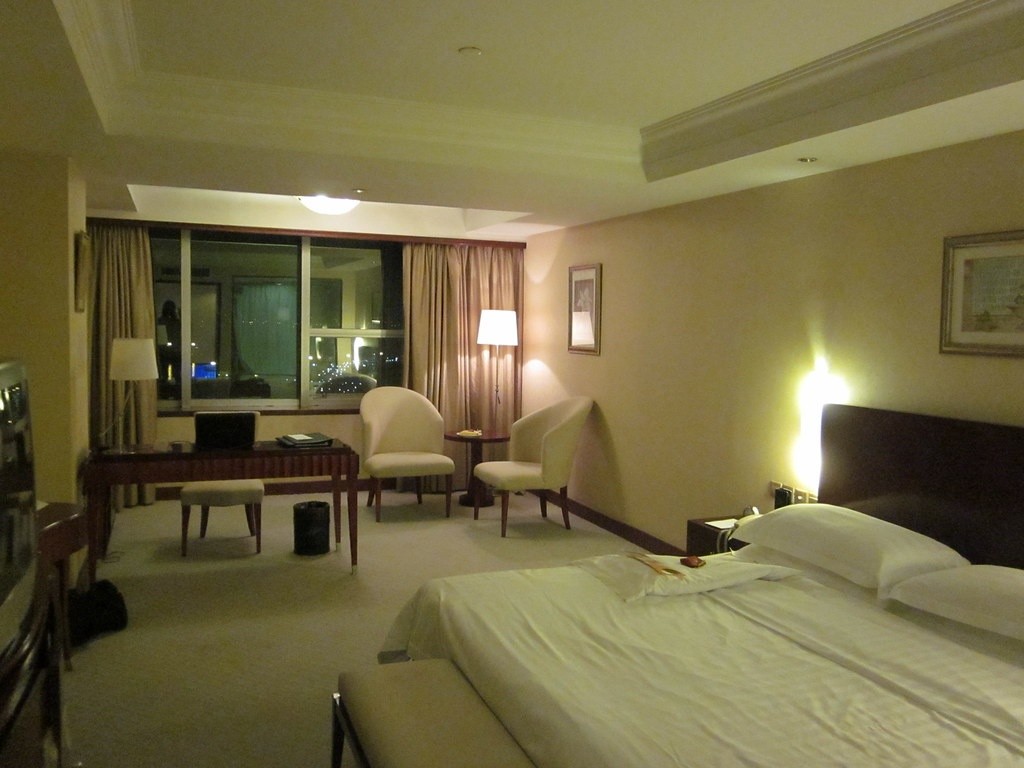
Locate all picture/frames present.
[939,230,1023,358]
[566,265,602,355]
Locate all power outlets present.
[770,481,793,501]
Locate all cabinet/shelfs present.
[0,502,85,768]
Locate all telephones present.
[733,513,764,527]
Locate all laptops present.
[194,413,255,449]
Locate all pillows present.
[885,557,1023,642]
[728,508,972,602]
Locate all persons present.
[158,300,181,384]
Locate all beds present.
[373,402,1024,768]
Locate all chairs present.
[360,386,455,523]
[471,399,596,539]
[177,410,263,557]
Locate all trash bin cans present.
[292,500,330,556]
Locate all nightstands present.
[686,515,748,561]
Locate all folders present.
[283,432,334,447]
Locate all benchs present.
[331,657,531,768]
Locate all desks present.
[444,429,511,507]
[77,438,357,586]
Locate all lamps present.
[88,338,158,454]
[477,309,518,495]
